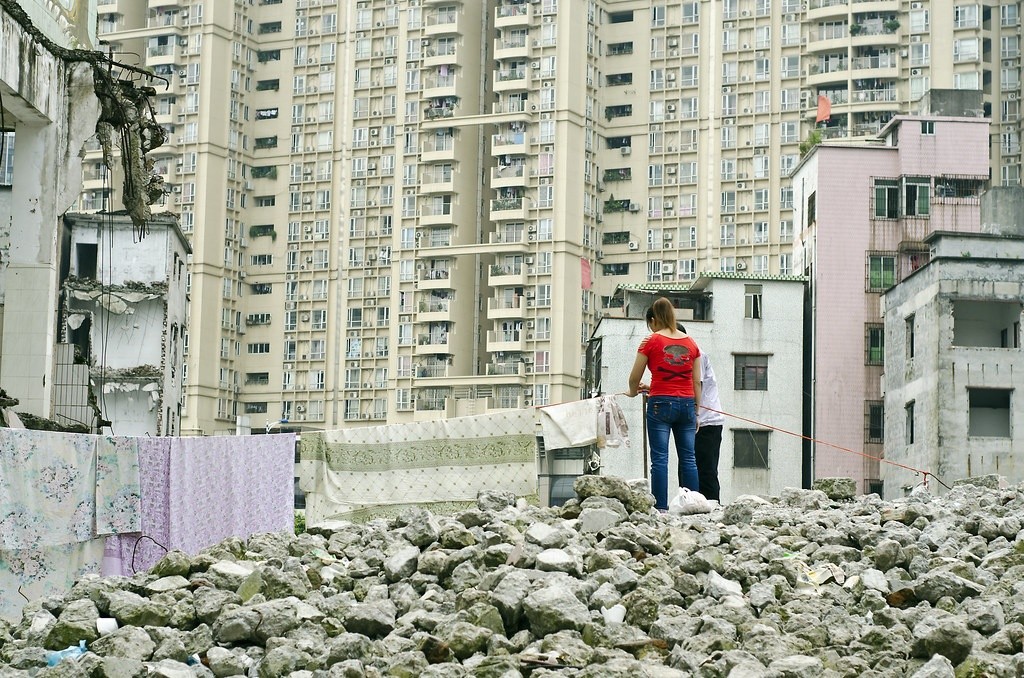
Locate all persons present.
[621,297,702,514]
[637,322,725,505]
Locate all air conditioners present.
[128,9,1023,422]
